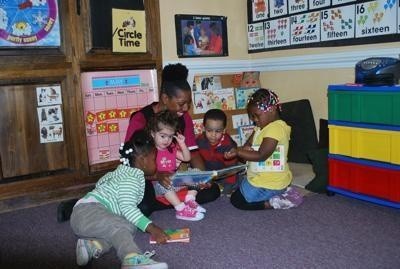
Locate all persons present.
[55,62,220,224]
[145,110,207,221]
[224,88,302,210]
[194,109,240,194]
[70,128,172,269]
[182,20,223,56]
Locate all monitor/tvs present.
[175,13,229,58]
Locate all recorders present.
[354,57,400,86]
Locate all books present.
[188,71,260,147]
[149,228,190,244]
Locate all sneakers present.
[122,255,168,268]
[77,239,98,265]
[184,199,206,213]
[176,207,204,221]
[57,198,77,222]
[269,187,302,209]
